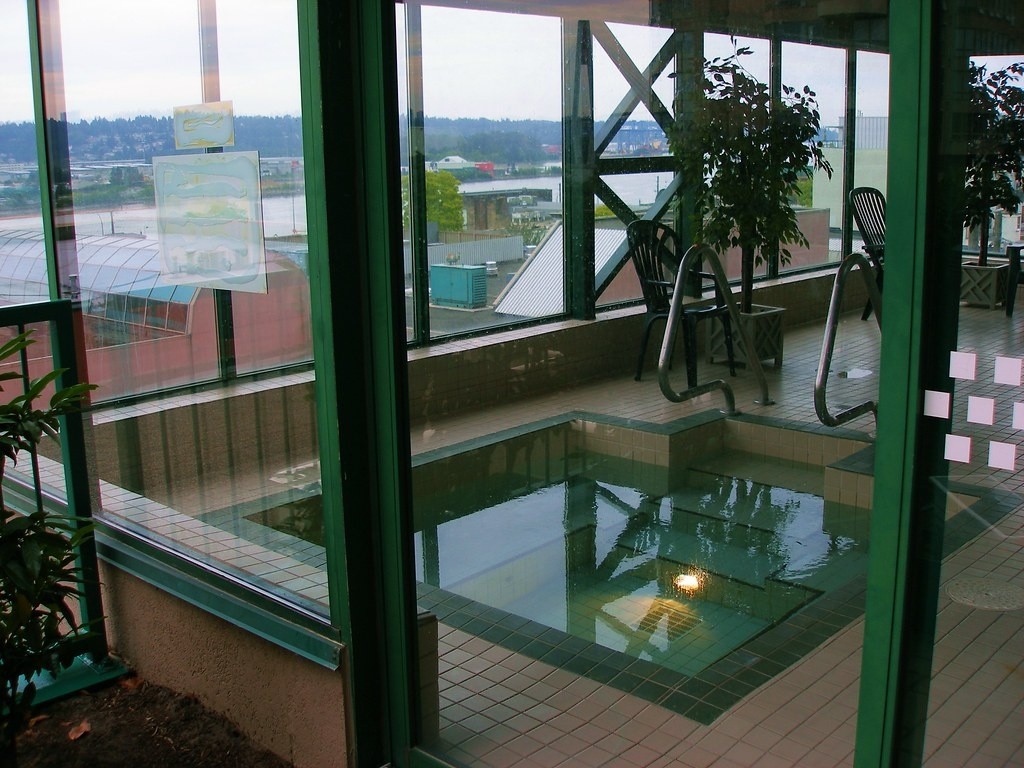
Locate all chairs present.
[1005,245,1024,318]
[848,186,886,320]
[626,219,738,390]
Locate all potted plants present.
[959,61,1024,311]
[666,29,835,371]
